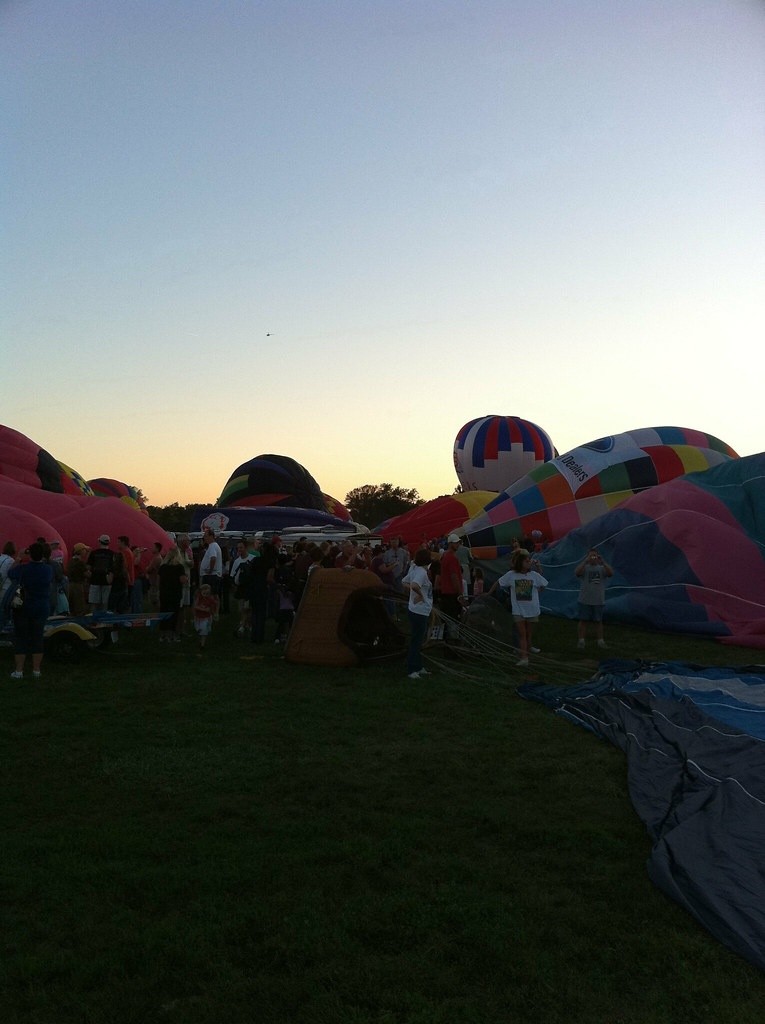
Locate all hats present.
[447,534,462,543]
[272,537,282,542]
[74,543,90,554]
[97,535,111,544]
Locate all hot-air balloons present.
[214,415,765,649]
[0,425,177,582]
[285,566,765,968]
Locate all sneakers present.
[417,667,433,675]
[408,672,421,679]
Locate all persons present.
[575,549,614,647]
[1,528,549,678]
[440,533,469,651]
[7,543,54,678]
[477,549,548,666]
[400,549,434,679]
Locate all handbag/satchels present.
[10,584,24,609]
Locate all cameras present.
[143,548,147,551]
[591,556,598,560]
[25,548,30,554]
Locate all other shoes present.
[249,627,252,631]
[10,670,24,678]
[596,639,607,649]
[577,638,585,648]
[528,647,541,654]
[274,639,279,646]
[515,657,529,666]
[237,626,245,633]
[32,670,42,676]
